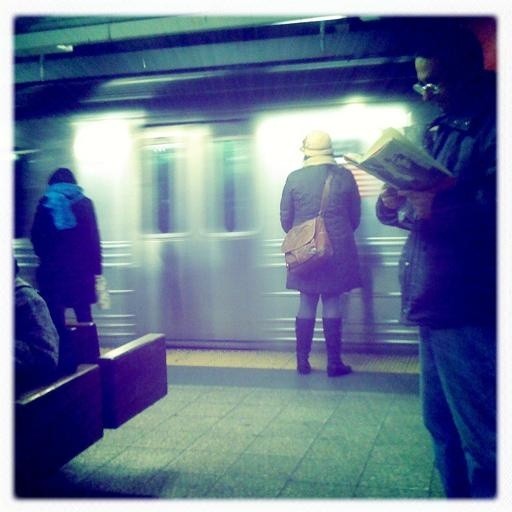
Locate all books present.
[343,126,457,195]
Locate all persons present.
[14,258,61,394]
[27,166,102,340]
[373,45,494,500]
[278,129,363,377]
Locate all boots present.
[323,317,351,377]
[295,318,315,374]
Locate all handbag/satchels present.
[281,217,333,275]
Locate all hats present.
[300,133,332,155]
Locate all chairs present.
[13,319,168,476]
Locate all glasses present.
[413,83,441,95]
